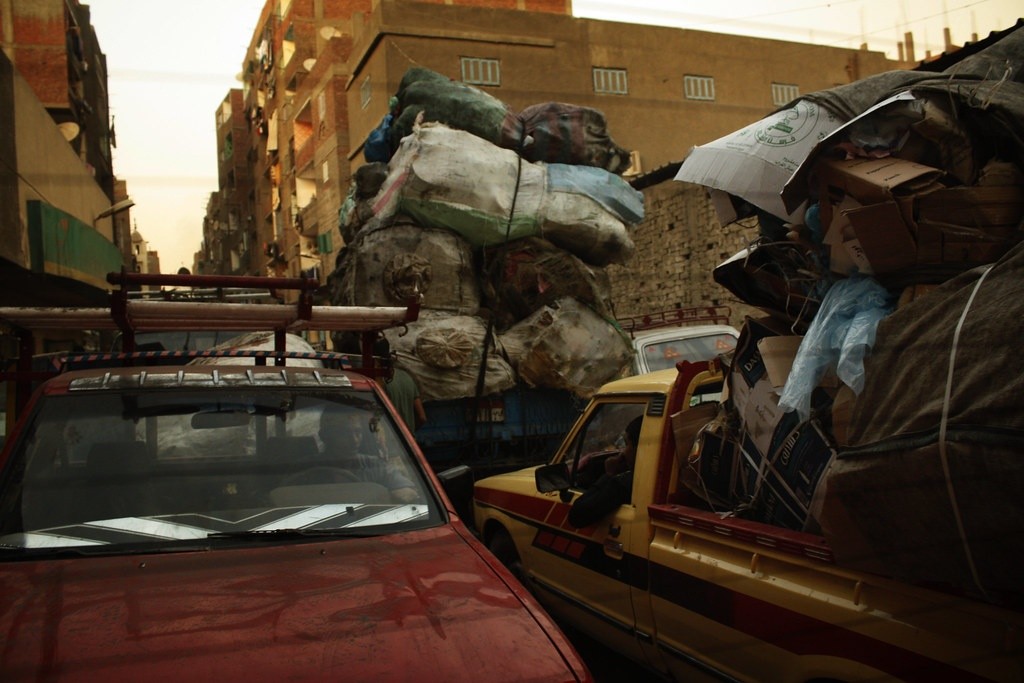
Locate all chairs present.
[241,435,319,510]
[69,440,172,524]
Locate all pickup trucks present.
[0,266,597,683]
[464,360,1023,681]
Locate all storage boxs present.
[666,70,1024,541]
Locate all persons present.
[290,402,418,505]
[568,416,644,529]
